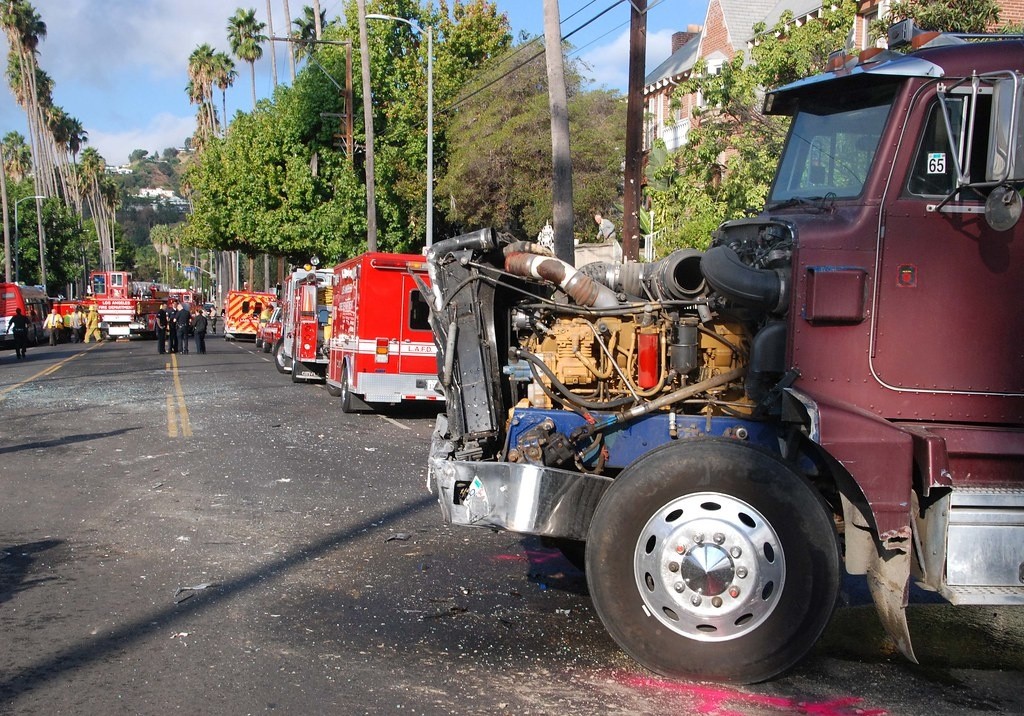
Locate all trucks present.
[426,20,1024,685]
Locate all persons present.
[6,308,32,359]
[155,299,217,355]
[43,304,102,347]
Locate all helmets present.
[89,305,95,310]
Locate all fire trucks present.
[274,252,447,413]
[220,291,278,342]
[0,271,199,346]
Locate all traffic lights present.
[177,262,182,272]
[157,272,164,284]
[214,279,216,287]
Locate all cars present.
[254,307,282,356]
[200,302,215,317]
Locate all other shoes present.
[22,351,25,357]
[160,350,206,354]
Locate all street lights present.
[365,14,433,248]
[15,196,46,281]
